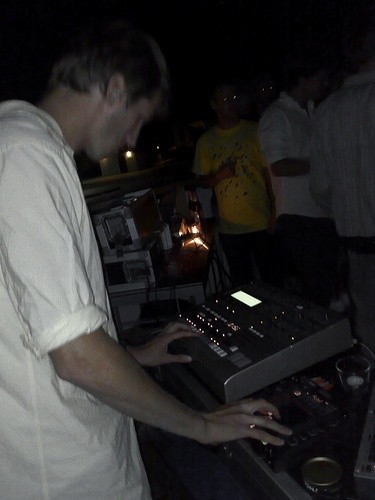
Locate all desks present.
[106,217,233,334]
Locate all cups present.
[334,356,371,400]
[301,456,343,498]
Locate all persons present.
[166,18,374,412]
[2,0,293,500]
